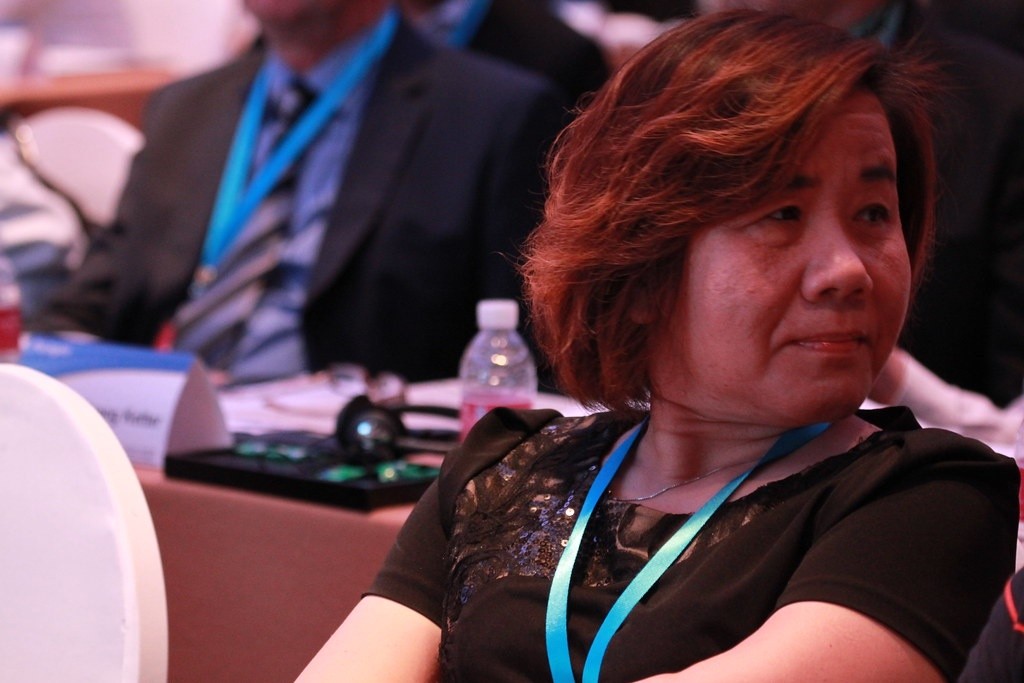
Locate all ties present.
[173,74,322,371]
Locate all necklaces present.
[612,456,760,502]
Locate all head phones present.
[335,395,463,466]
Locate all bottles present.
[458,298,537,444]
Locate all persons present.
[699,0,1024,451]
[47,1,700,385]
[291,9,1021,683]
